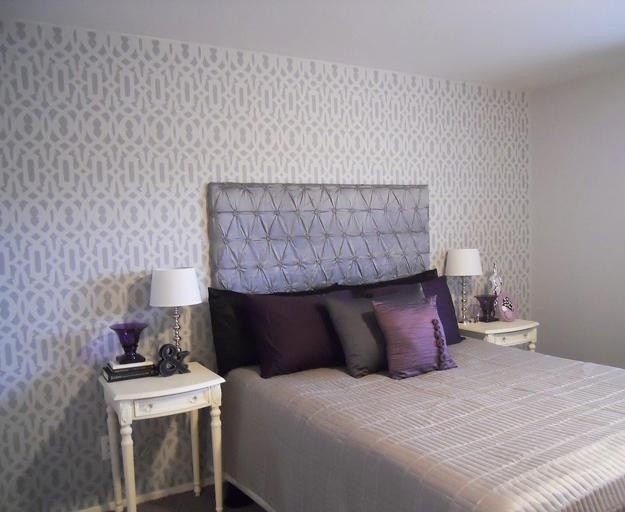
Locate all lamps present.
[148,266,210,371]
[443,247,486,326]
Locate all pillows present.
[207,267,468,382]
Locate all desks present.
[95,359,228,471]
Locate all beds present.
[204,181,625,471]
